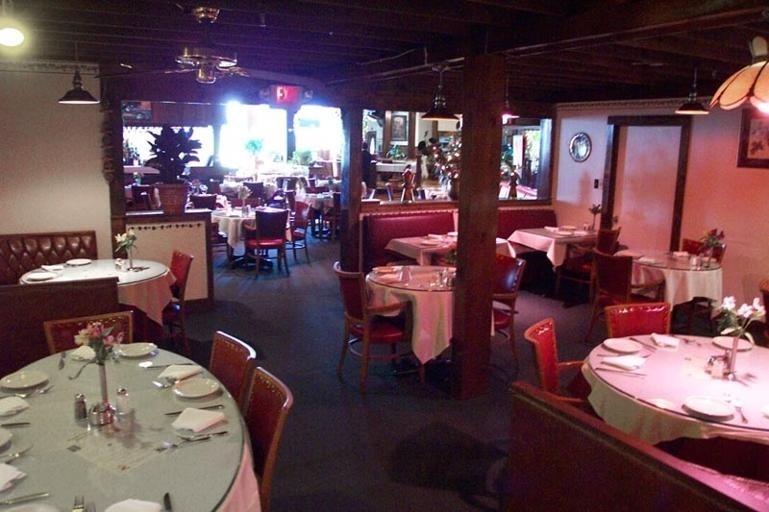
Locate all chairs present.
[126,157,519,281]
[1,226,195,358]
[209,328,257,417]
[524,317,588,408]
[329,200,725,386]
[241,365,294,512]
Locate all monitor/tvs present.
[270,83,304,109]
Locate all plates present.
[28,273,55,280]
[119,341,158,358]
[173,378,220,398]
[67,259,92,266]
[603,336,643,353]
[713,335,752,351]
[372,266,400,274]
[102,499,158,512]
[0,370,58,511]
[682,395,736,419]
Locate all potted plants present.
[144,121,203,215]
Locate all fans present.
[95,5,327,90]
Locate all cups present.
[428,269,454,290]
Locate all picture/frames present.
[389,115,407,141]
[737,107,769,168]
[567,130,593,162]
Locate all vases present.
[727,336,741,381]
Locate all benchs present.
[507,379,768,510]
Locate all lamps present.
[707,34,769,116]
[501,74,521,127]
[58,35,101,106]
[676,68,709,116]
[420,62,459,124]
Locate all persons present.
[361,142,371,187]
[417,137,444,162]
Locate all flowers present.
[713,292,767,341]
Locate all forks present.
[171,429,227,441]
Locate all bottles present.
[399,266,411,284]
[73,393,87,421]
[114,387,132,416]
[115,257,122,271]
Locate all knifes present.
[142,363,193,369]
[57,352,67,369]
[163,492,172,511]
[595,368,647,377]
[164,404,224,416]
